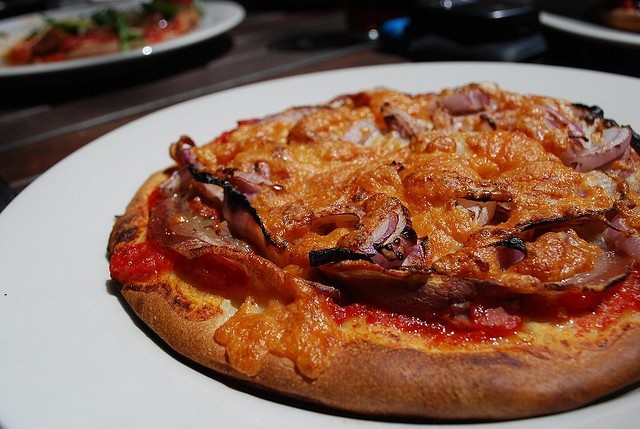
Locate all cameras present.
[380,0,549,65]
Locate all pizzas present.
[107,81,637,420]
[0,0,204,64]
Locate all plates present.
[0,0,247,75]
[0,60,640,427]
